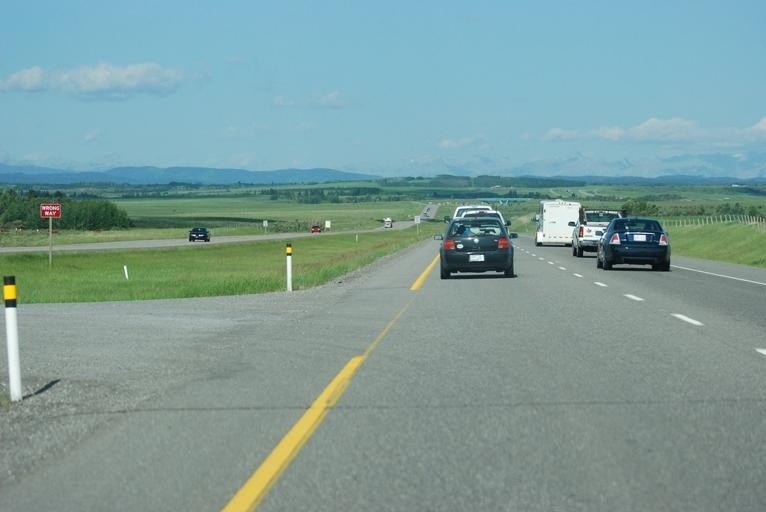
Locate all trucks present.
[385,218,392,228]
[534,200,581,246]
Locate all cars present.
[311,226,321,233]
[597,217,670,269]
[434,205,518,278]
[189,228,210,242]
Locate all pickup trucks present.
[572,210,622,256]
